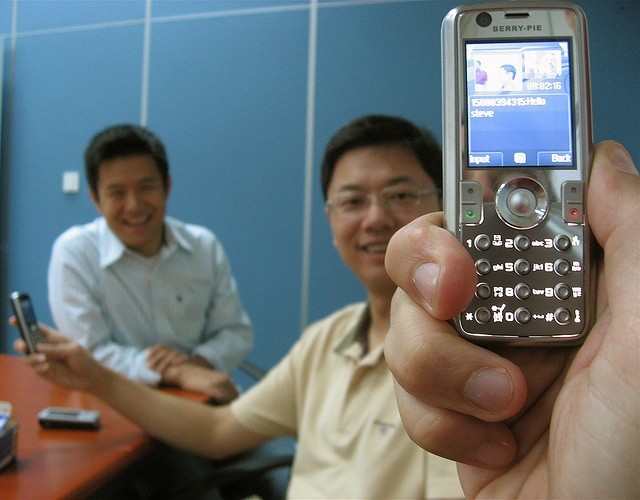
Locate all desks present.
[0,351,213,500]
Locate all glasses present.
[326,187,438,217]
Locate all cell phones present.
[440,0,598,348]
[10,290,46,354]
[37,407,102,430]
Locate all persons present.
[499,64,518,91]
[9,114,465,500]
[383,139,640,499]
[46,122,298,500]
[475,60,487,85]
